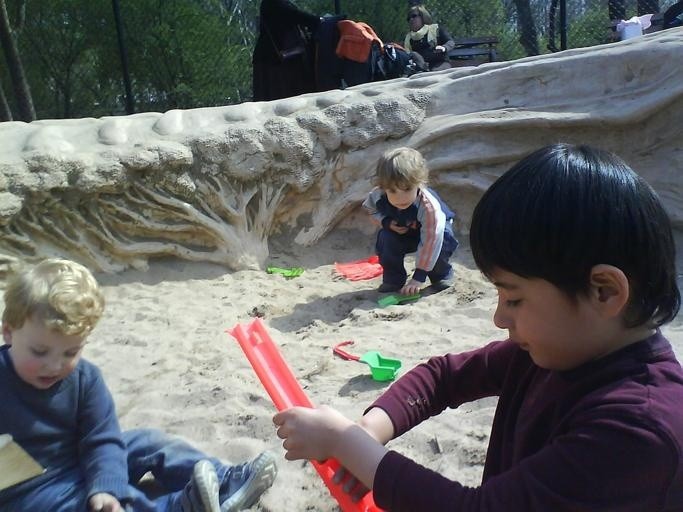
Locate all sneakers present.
[377,274,407,292]
[434,271,455,288]
[181,459,220,511]
[220,451,277,511]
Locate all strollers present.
[320,12,428,85]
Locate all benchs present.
[605,13,665,42]
[445,36,500,68]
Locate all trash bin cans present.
[620,19,643,40]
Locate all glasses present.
[407,13,421,21]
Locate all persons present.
[403,3,455,74]
[359,143,459,293]
[270,141,680,511]
[1,258,279,512]
